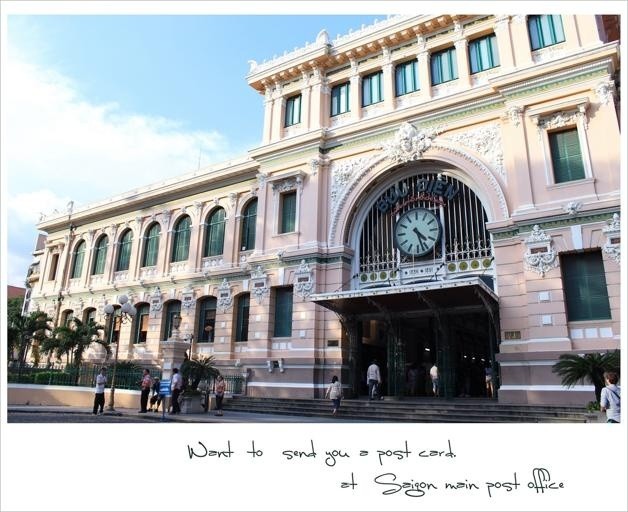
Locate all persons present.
[366,359,382,401]
[215,375,225,416]
[382,361,495,398]
[91,367,107,416]
[147,376,165,412]
[137,369,151,413]
[324,375,343,417]
[599,371,619,423]
[170,368,182,414]
[197,377,209,412]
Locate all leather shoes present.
[138,409,147,413]
[169,410,181,415]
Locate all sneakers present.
[214,413,224,416]
[92,413,104,416]
[147,409,159,412]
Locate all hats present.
[154,376,160,381]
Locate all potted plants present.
[550,349,620,423]
[178,352,220,413]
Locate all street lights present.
[104,295,137,413]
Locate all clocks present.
[394,208,441,258]
